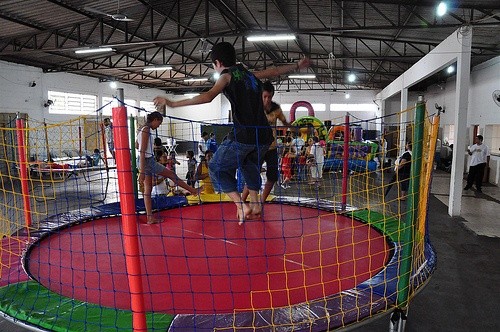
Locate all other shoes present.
[286,186,291,188]
[477,188,482,192]
[281,185,286,189]
[464,185,471,190]
[400,197,406,200]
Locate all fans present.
[492,90,500,107]
[83,0,135,21]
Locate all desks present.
[163,144,179,156]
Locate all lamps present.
[73,48,117,54]
[142,66,175,71]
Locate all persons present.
[394,142,412,201]
[464,135,491,193]
[237,80,300,217]
[275,131,324,190]
[137,110,218,224]
[83,149,105,167]
[100,118,115,159]
[152,39,314,225]
